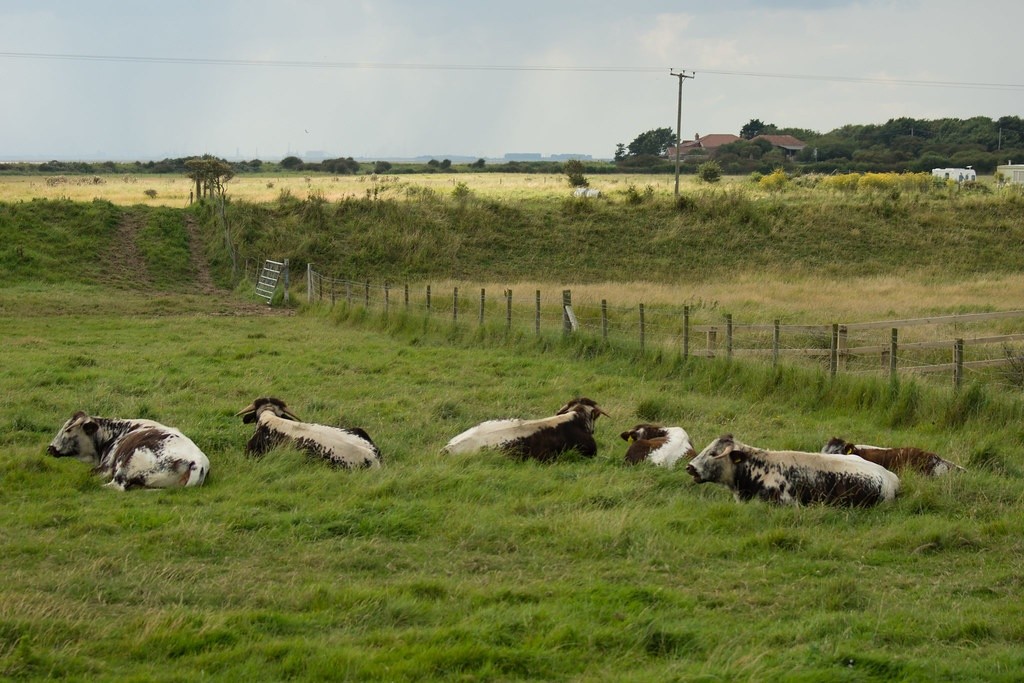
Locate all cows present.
[47,411,210,491]
[685,433,902,511]
[819,436,967,477]
[440,396,610,466]
[236,396,384,474]
[619,422,696,470]
